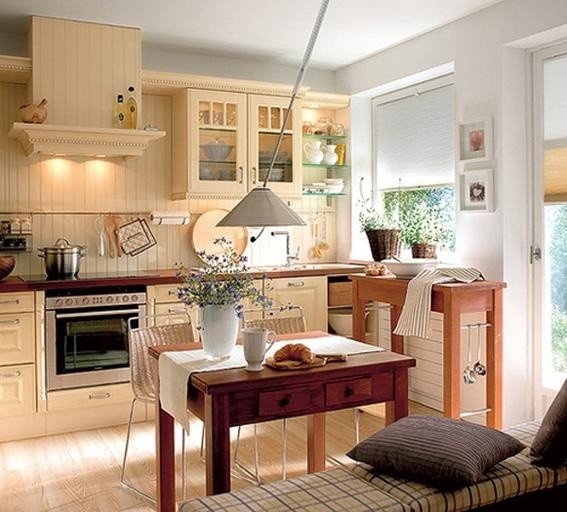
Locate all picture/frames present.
[455,117,493,164]
[456,169,494,213]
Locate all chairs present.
[120,311,261,507]
[235,305,359,482]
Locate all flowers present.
[167,237,296,319]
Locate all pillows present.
[344,413,529,488]
[529,378,567,468]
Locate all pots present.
[37,238,88,281]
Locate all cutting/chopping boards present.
[265,353,347,372]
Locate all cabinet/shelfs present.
[264,276,328,331]
[171,88,303,201]
[0,292,37,417]
[147,280,264,339]
[47,383,146,436]
[303,135,349,196]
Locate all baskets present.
[411,243,436,259]
[366,228,401,262]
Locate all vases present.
[198,306,238,361]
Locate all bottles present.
[10,218,21,236]
[337,143,346,166]
[21,217,32,235]
[0,220,10,236]
[124,85,139,131]
[113,94,127,129]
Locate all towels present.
[393,265,487,341]
[265,344,347,372]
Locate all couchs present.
[178,419,567,511]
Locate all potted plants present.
[356,176,404,260]
[401,194,455,258]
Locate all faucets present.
[272,229,300,264]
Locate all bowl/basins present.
[200,143,236,160]
[259,168,284,181]
[328,308,370,337]
[324,178,345,195]
[380,258,441,279]
[1,255,17,282]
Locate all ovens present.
[44,285,147,392]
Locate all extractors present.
[8,14,167,161]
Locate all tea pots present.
[321,144,339,165]
[303,141,323,165]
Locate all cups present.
[199,166,216,180]
[203,110,220,124]
[218,168,236,180]
[214,111,229,125]
[242,328,276,372]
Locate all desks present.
[349,274,507,431]
[148,330,416,512]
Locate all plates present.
[258,151,289,161]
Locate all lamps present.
[213,1,330,227]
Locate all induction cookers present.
[18,268,161,283]
